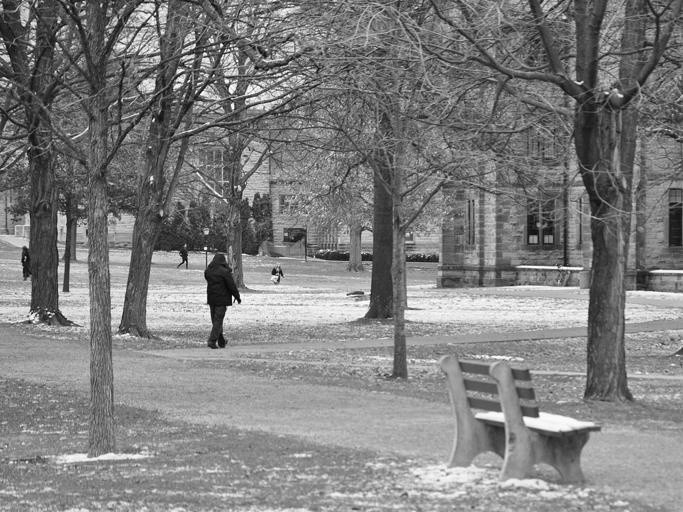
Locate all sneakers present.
[208,342,218,349]
[219,337,229,348]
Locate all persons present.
[203,252,240,349]
[178,245,188,269]
[271,264,285,283]
[21,246,31,280]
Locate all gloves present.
[232,295,242,305]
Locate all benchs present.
[436,353,601,484]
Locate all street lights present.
[202,226,210,267]
[303,222,308,261]
